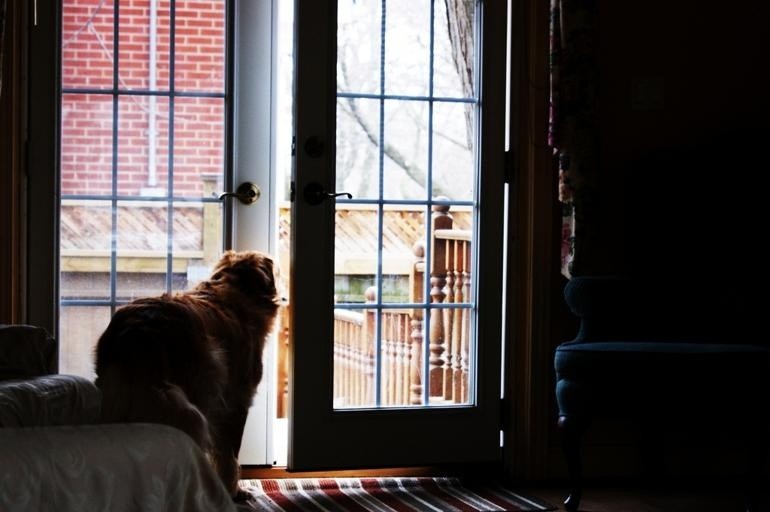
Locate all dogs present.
[90,249,280,504]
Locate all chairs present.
[550,276,767,511]
